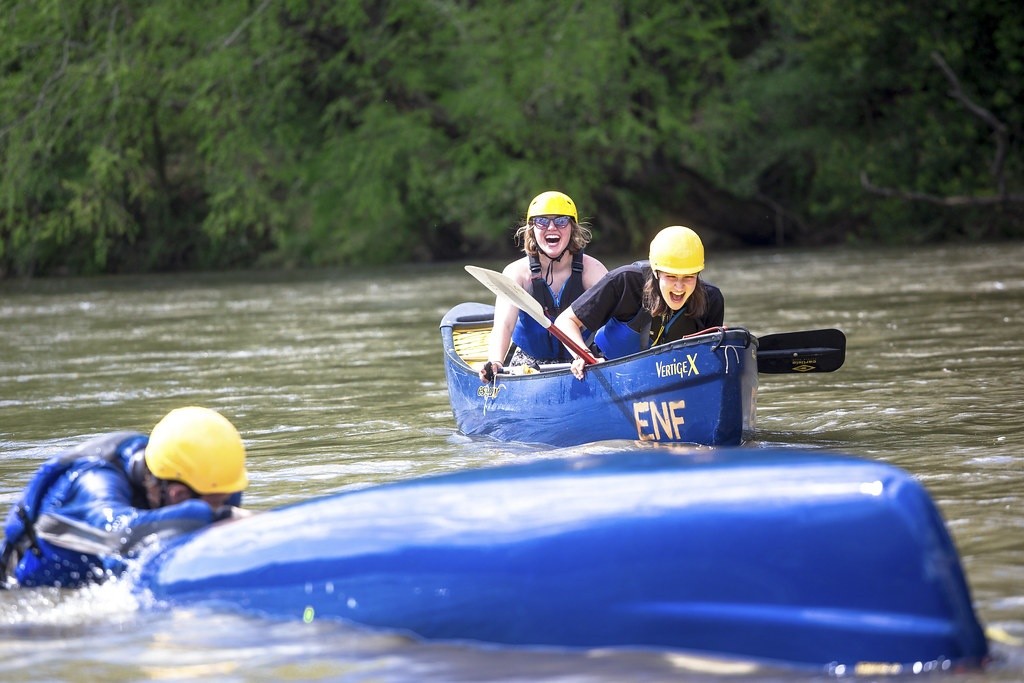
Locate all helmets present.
[144,406,249,496]
[649,226,705,279]
[527,191,578,226]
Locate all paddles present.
[463,262,598,366]
[497,327,848,376]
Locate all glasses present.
[532,216,571,231]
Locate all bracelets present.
[576,349,591,360]
[493,361,503,367]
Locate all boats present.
[439,301,760,449]
[118,444,992,669]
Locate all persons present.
[554,225,725,379]
[6,406,252,588]
[479,191,609,382]
